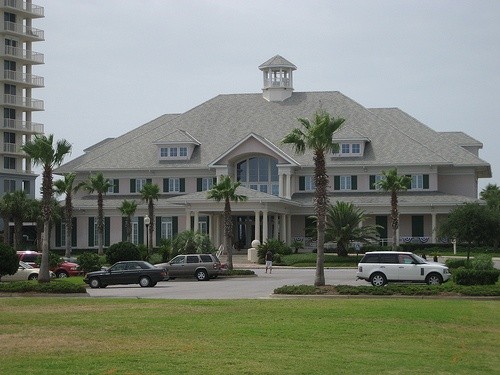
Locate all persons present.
[265,249,273,274]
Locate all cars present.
[1,261,56,281]
[83,261,168,288]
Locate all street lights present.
[144,215,150,249]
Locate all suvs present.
[153,254,221,281]
[356,252,452,287]
[16,251,81,278]
